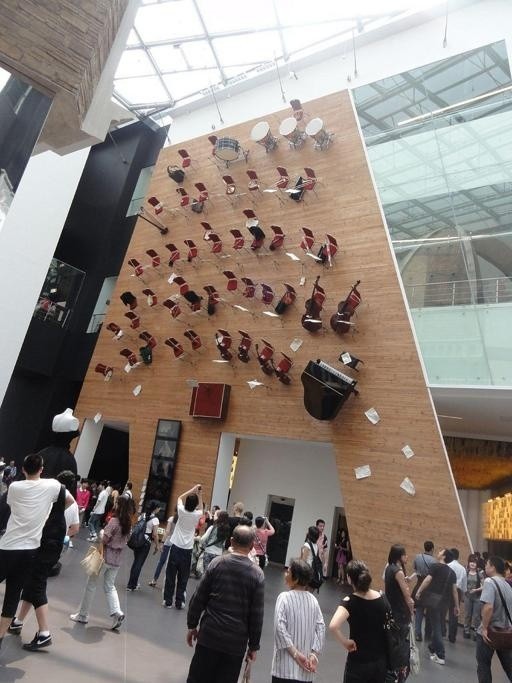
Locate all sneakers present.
[429,654,445,667]
[22,631,51,650]
[161,598,173,609]
[126,583,140,592]
[175,600,185,610]
[6,617,22,635]
[70,612,89,624]
[85,521,97,542]
[111,611,125,629]
[416,632,478,641]
[68,541,74,548]
[149,579,157,587]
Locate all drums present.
[279,117,303,149]
[215,139,239,160]
[252,122,277,151]
[306,118,328,146]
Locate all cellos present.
[301,276,322,331]
[271,358,292,385]
[237,340,249,363]
[330,280,360,333]
[215,334,232,361]
[255,344,273,375]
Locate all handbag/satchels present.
[264,552,271,567]
[409,646,419,677]
[80,544,105,581]
[196,551,203,574]
[486,626,512,651]
[419,591,445,609]
[38,502,66,567]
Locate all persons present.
[301,519,349,584]
[272,557,326,682]
[186,523,266,682]
[1,455,134,649]
[328,540,512,682]
[126,484,275,608]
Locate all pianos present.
[301,359,359,421]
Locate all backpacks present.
[127,513,158,552]
[307,542,323,588]
[384,618,413,670]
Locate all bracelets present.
[293,649,297,658]
[308,652,319,662]
